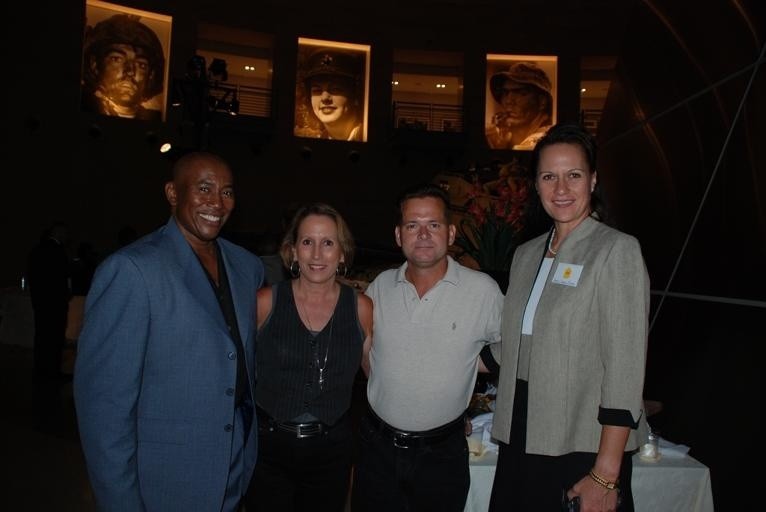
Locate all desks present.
[464,436,715,512]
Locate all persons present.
[255,199,306,287]
[479,123,653,512]
[26,218,164,434]
[350,183,507,512]
[252,200,375,511]
[297,45,369,141]
[485,61,556,154]
[85,9,166,124]
[72,149,364,509]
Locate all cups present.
[640,435,659,457]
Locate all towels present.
[658,437,690,459]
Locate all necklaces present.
[296,273,337,392]
[548,226,560,256]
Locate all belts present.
[261,410,330,440]
[366,402,463,454]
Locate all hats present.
[298,50,368,91]
[489,64,554,116]
[59,17,164,82]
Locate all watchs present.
[587,467,621,492]
